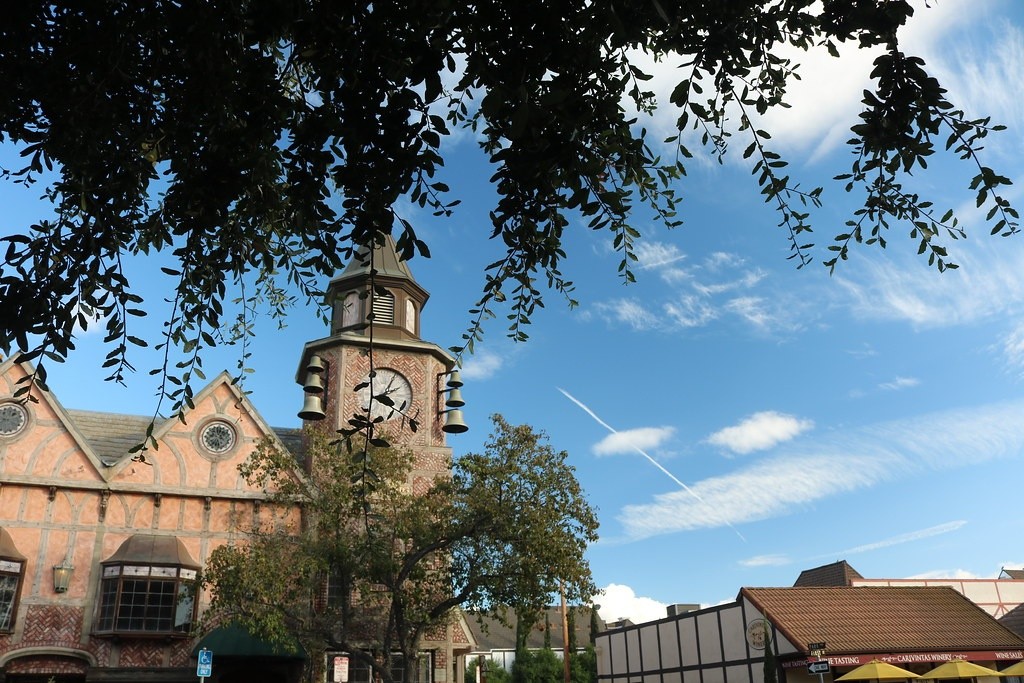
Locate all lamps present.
[53,553,76,592]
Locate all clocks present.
[359,364,416,422]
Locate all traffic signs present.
[806,660,831,676]
